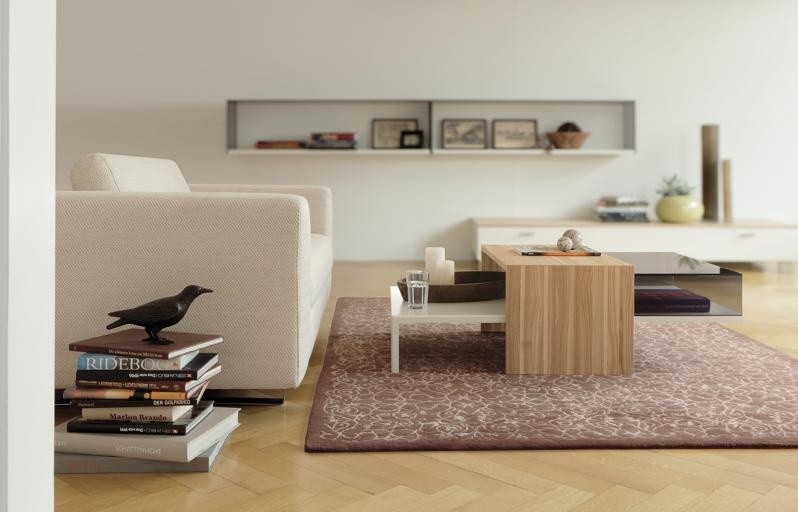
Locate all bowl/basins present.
[544,132,591,149]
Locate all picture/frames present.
[370,119,537,149]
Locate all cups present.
[405,270,429,313]
[425,246,455,286]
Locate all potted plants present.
[654,172,705,222]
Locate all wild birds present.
[106,285,213,344]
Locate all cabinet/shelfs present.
[472,220,798,275]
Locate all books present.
[54,329,242,473]
[515,246,601,256]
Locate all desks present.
[390,243,742,378]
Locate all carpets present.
[306,295,798,449]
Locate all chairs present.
[55,153,335,407]
